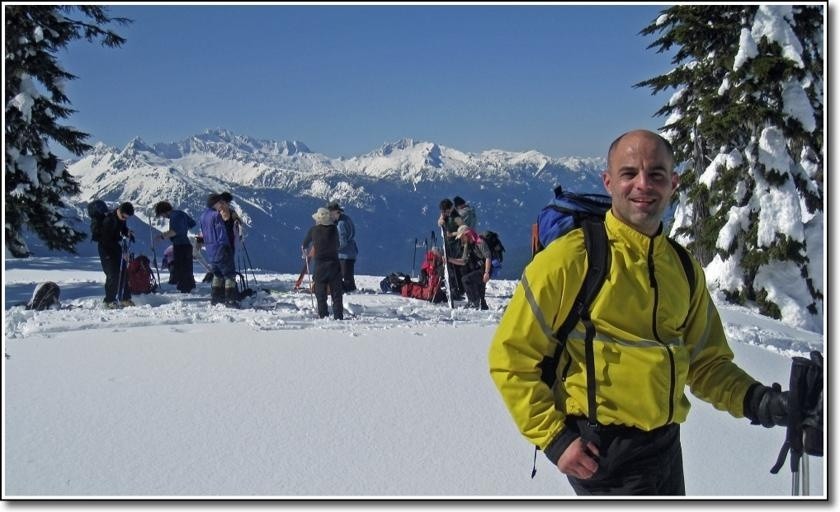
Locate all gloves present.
[743,380,793,426]
[800,348,824,458]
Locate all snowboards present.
[440,223,453,309]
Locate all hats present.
[312,207,332,226]
[207,194,224,208]
[454,196,465,207]
[327,203,344,212]
[455,225,471,240]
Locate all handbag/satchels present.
[379,249,447,303]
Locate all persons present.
[301,207,345,320]
[436,198,463,301]
[89,188,241,309]
[486,129,793,494]
[441,224,490,310]
[453,197,476,226]
[326,202,357,290]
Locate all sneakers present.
[108,300,136,309]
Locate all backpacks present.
[25,280,61,311]
[477,231,506,262]
[126,252,158,294]
[530,184,612,266]
[87,200,111,242]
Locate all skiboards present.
[115,231,136,308]
[294,256,311,294]
[429,231,438,301]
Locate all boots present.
[211,286,240,309]
